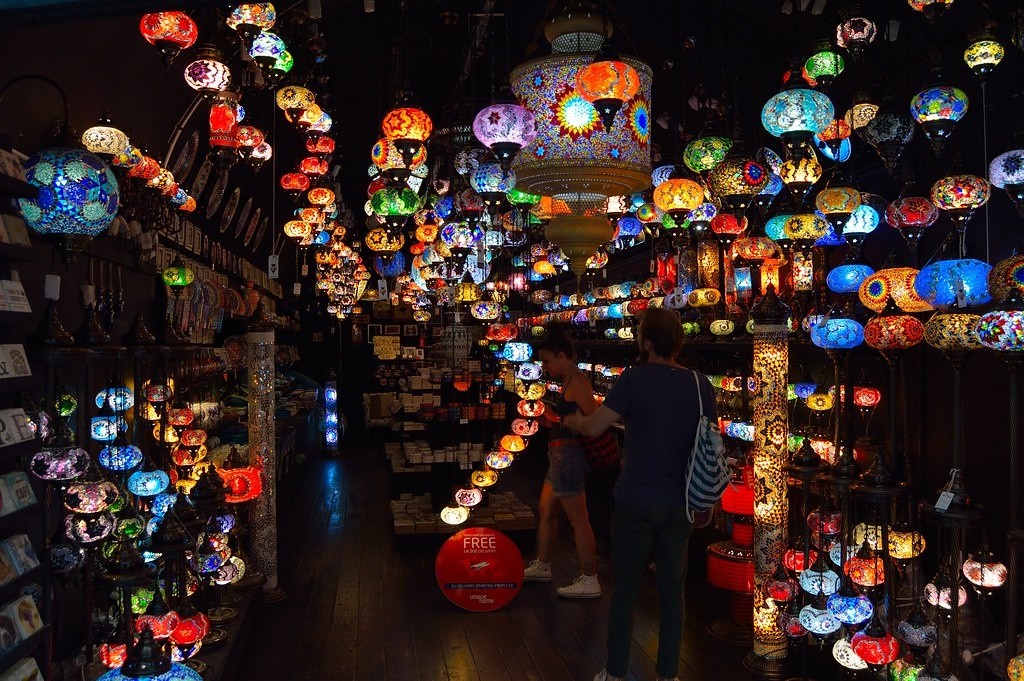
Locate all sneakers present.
[523,557,552,582]
[556,573,601,598]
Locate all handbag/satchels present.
[581,429,622,471]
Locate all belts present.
[548,438,583,448]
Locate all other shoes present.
[656,674,680,681]
[593,668,622,681]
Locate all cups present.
[404,441,483,464]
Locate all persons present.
[559,308,720,681]
[581,434,621,533]
[523,326,600,597]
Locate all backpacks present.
[685,369,738,530]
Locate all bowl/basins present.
[276,384,320,420]
[421,402,508,420]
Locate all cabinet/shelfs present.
[560,329,880,548]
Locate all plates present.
[170,128,269,253]
[186,278,252,317]
[223,334,249,367]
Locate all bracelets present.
[560,416,566,428]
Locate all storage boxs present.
[368,392,392,419]
